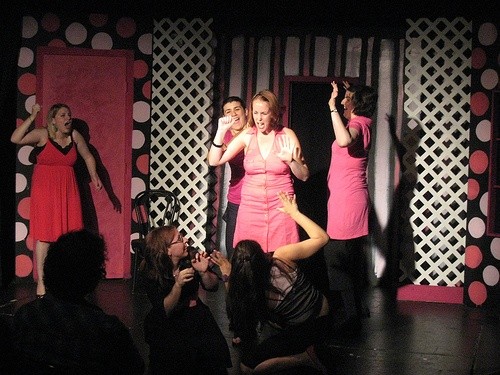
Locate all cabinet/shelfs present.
[486,90,500,238]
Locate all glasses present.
[168,233,183,245]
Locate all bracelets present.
[330,108,338,112]
[212,142,222,148]
[221,275,232,282]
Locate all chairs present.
[132,189,178,291]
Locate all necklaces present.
[173,274,177,277]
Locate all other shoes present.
[36,285,47,300]
[333,317,364,336]
[306,344,337,375]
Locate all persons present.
[207,88,309,256]
[9,228,146,375]
[324,80,379,336]
[132,226,233,375]
[220,96,248,260]
[210,192,334,375]
[10,102,102,300]
